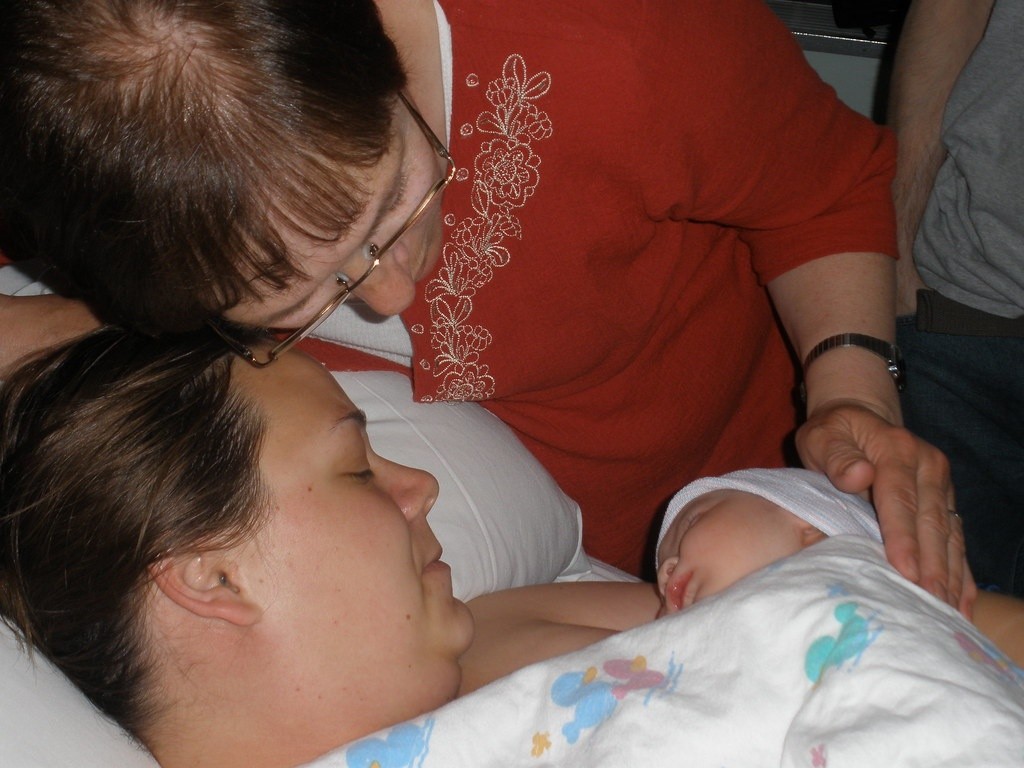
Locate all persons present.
[656,468,1024,768]
[889,0,1024,599]
[0,0,979,607]
[0,316,1024,768]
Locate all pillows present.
[1,359,587,766]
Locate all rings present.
[948,511,962,524]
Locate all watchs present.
[799,333,902,399]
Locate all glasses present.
[196,91,457,370]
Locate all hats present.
[655,466,884,569]
